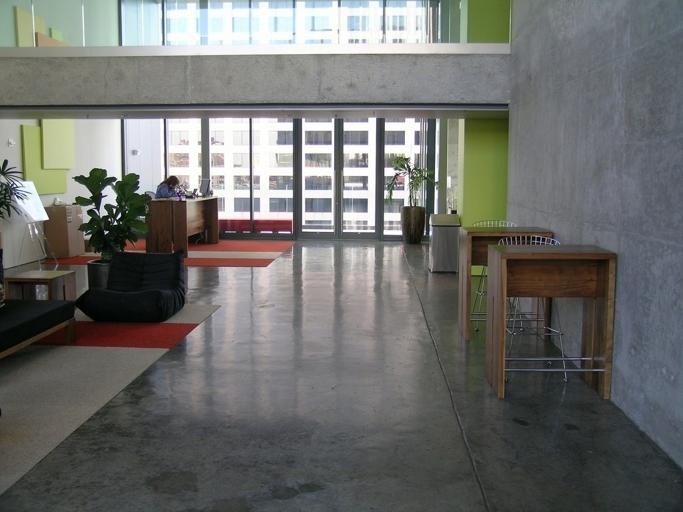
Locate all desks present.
[145,194,219,258]
[484,244,616,403]
[4,270,75,344]
[455,226,554,343]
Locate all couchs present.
[75,251,187,325]
[0,250,77,360]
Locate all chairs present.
[472,218,525,335]
[496,233,569,382]
[144,190,155,199]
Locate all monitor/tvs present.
[200,179,210,197]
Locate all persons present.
[154,175,180,198]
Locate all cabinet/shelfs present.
[44,204,84,257]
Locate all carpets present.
[40,232,294,268]
[0,301,220,493]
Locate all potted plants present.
[385,153,439,244]
[70,167,151,292]
